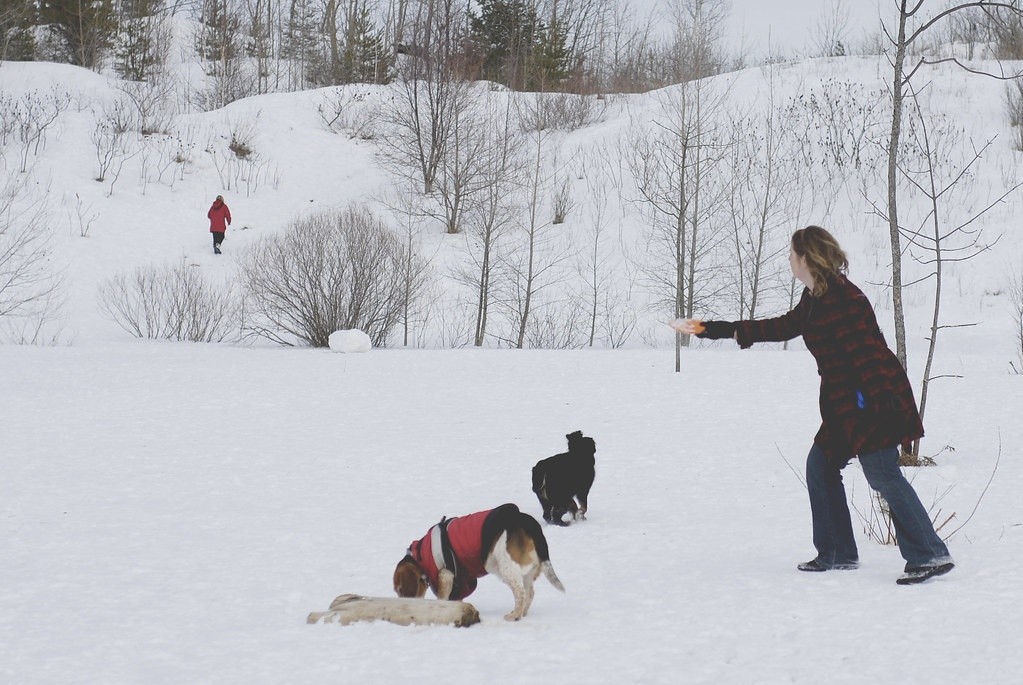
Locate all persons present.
[207,194,231,257]
[694,225,956,584]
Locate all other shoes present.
[797,560,826,571]
[897,563,954,585]
[214,247,222,253]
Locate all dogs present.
[392,503,565,623]
[532,429,596,527]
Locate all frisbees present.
[670,318,706,333]
